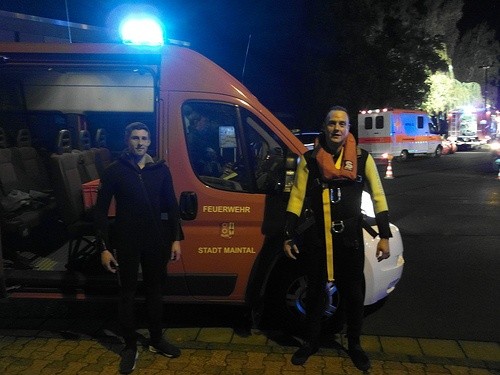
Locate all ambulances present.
[358,108,444,162]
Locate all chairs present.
[0,104,241,271]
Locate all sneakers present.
[348,344,369,370]
[291,341,319,365]
[149,338,180,358]
[119,345,139,372]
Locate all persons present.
[282,105,392,370]
[93,123,185,375]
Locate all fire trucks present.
[0,8,405,339]
